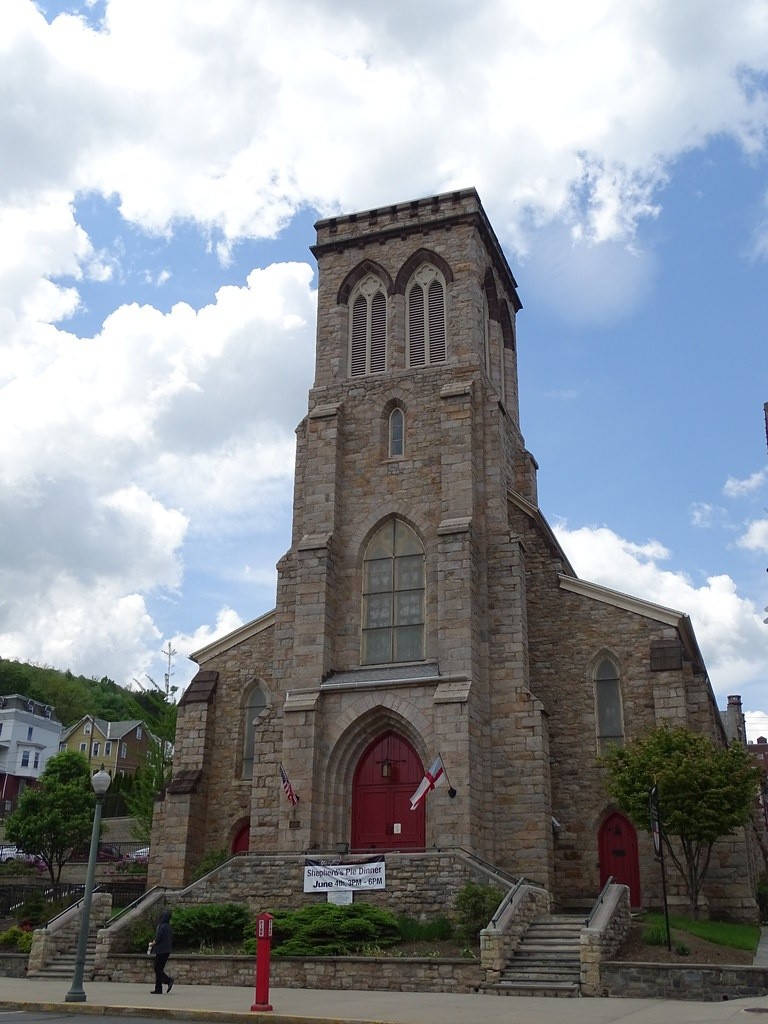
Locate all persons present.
[149,911,174,994]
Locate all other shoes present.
[151,986,162,994]
[167,979,174,993]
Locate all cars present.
[69,843,124,863]
[132,847,151,862]
[0,848,43,865]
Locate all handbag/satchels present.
[148,942,157,954]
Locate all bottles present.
[147,942,153,955]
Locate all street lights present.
[63,763,113,1003]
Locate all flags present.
[280,767,298,806]
[410,755,445,810]
